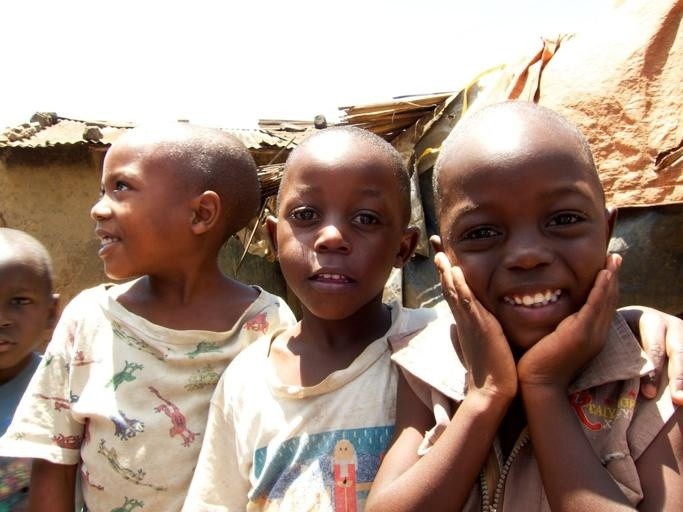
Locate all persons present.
[1,123,299,512]
[1,227,82,512]
[364,99,683,512]
[182,125,683,512]
